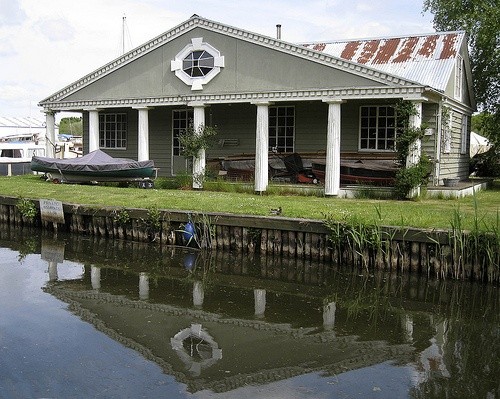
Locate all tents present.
[29,148,156,173]
